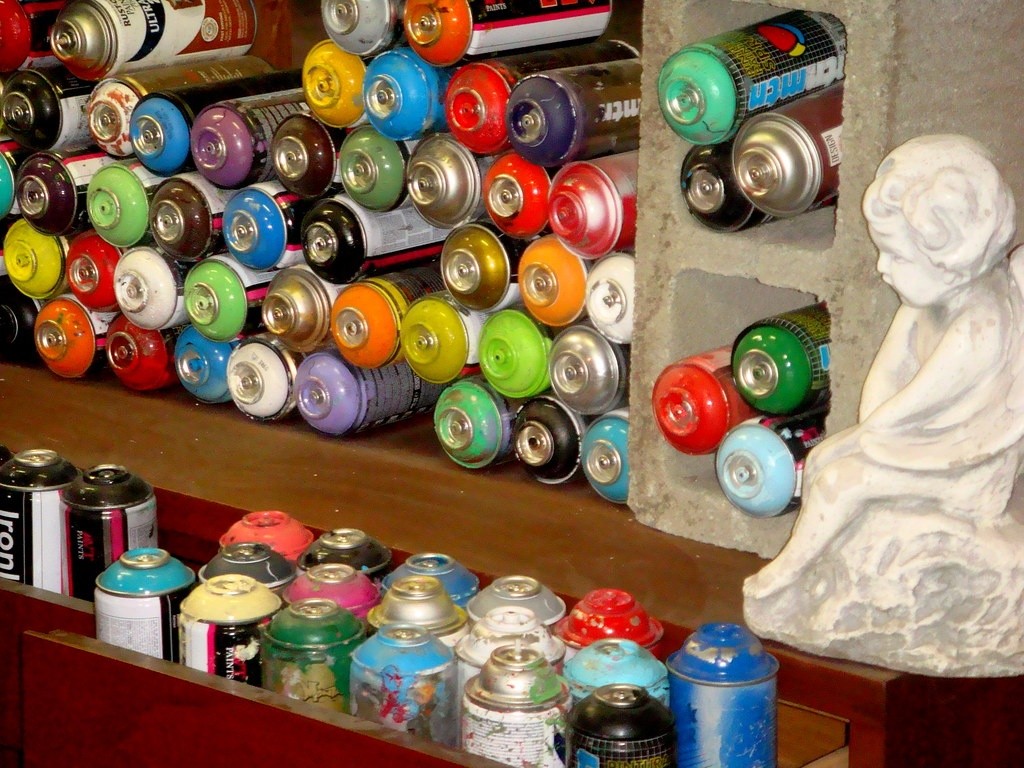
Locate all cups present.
[655,10,845,236]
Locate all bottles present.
[260,0,628,506]
[455,646,571,768]
[365,552,480,642]
[0,0,307,422]
[260,598,365,718]
[454,573,569,664]
[349,625,459,749]
[199,510,313,594]
[0,447,158,603]
[1,316,2,317]
[180,573,282,686]
[92,547,197,665]
[555,587,779,768]
[651,303,834,521]
[286,525,389,615]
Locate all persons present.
[743,127,1024,601]
